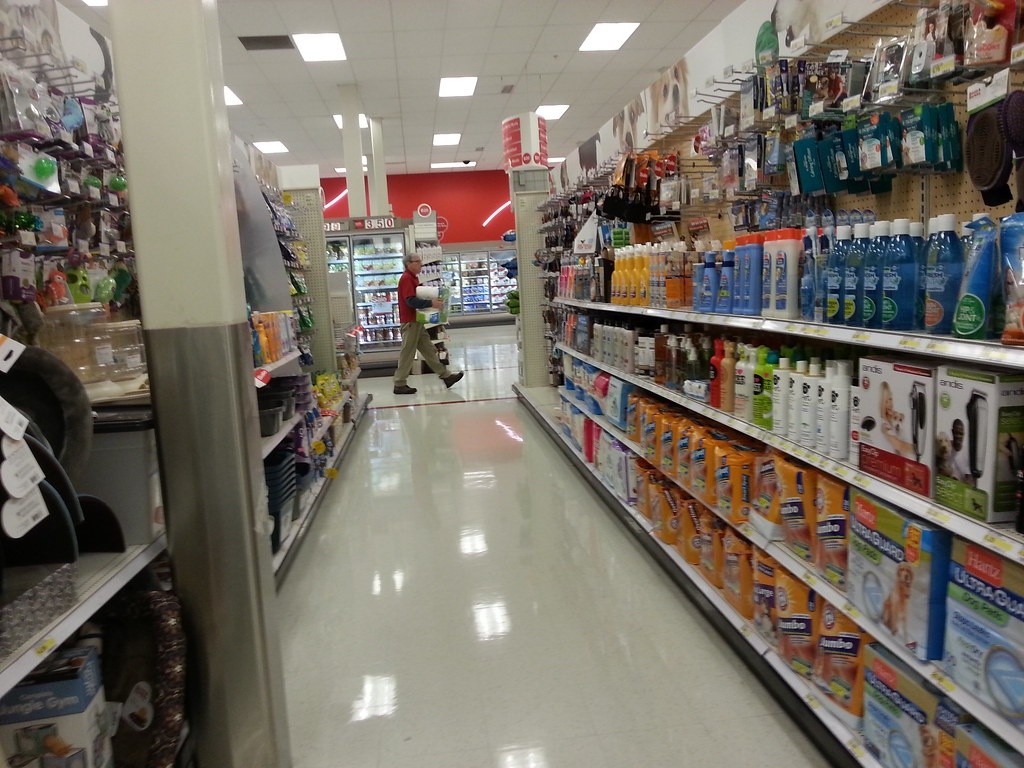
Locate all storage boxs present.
[933,363,1024,524]
[0,677,101,768]
[793,100,963,197]
[955,725,1024,768]
[943,538,1023,733]
[266,449,296,553]
[862,643,976,768]
[17,643,114,768]
[858,353,933,500]
[847,485,949,661]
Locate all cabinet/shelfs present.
[411,223,447,374]
[0,0,373,768]
[553,0,1024,768]
[443,248,518,319]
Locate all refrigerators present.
[324,231,415,351]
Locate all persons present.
[875,381,917,458]
[392,252,463,394]
[947,419,978,489]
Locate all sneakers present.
[443,371,463,388]
[393,384,417,394]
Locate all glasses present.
[409,260,422,263]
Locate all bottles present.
[45,300,148,384]
